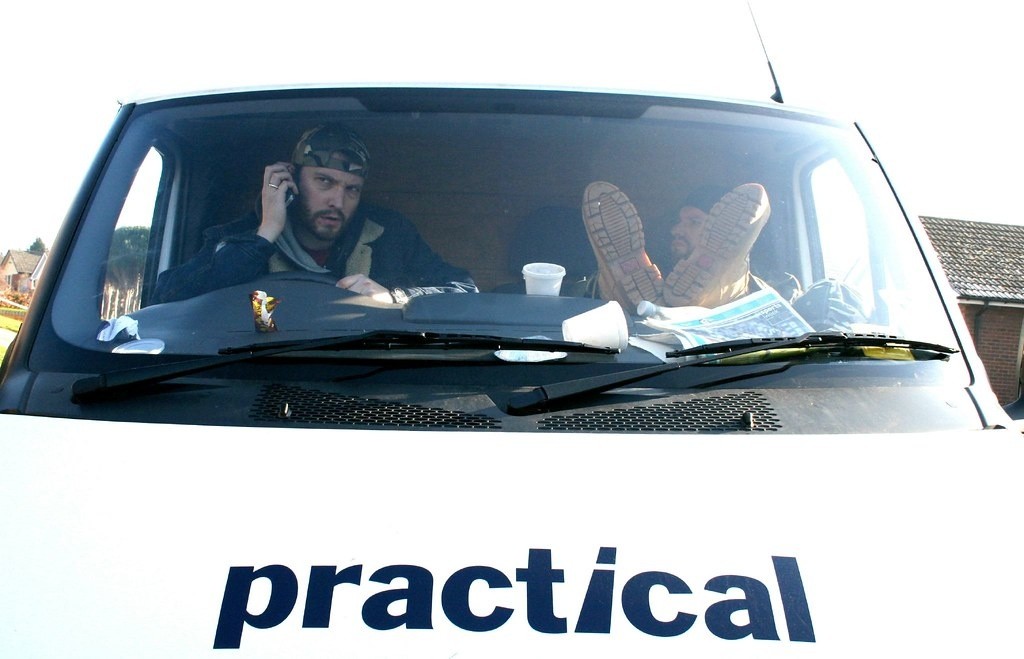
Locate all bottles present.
[637,301,710,322]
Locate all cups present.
[521,263,566,296]
[562,300,629,351]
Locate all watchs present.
[390,287,408,304]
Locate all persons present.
[582,180,772,313]
[150,123,480,306]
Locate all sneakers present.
[662,181,772,309]
[580,180,669,311]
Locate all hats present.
[684,184,731,216]
[291,120,372,178]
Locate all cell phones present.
[285,188,294,207]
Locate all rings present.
[269,183,278,189]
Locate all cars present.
[1,81,1024,655]
